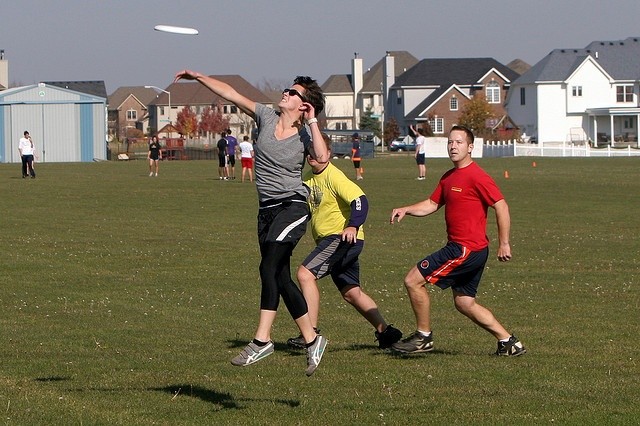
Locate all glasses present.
[283,88,305,102]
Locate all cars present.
[388,136,416,152]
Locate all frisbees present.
[153,24,199,36]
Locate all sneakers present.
[231,341,274,366]
[422,176,425,179]
[393,331,435,353]
[287,334,304,348]
[492,335,526,357]
[304,336,328,377]
[414,177,421,180]
[374,326,403,347]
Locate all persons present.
[18,131,38,179]
[286,133,402,353]
[174,70,332,378]
[227,129,239,180]
[391,127,525,359]
[217,132,230,181]
[239,136,255,182]
[147,136,162,177]
[350,135,365,181]
[409,125,427,181]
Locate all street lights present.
[144,85,171,126]
[379,79,385,152]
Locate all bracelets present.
[306,117,318,125]
